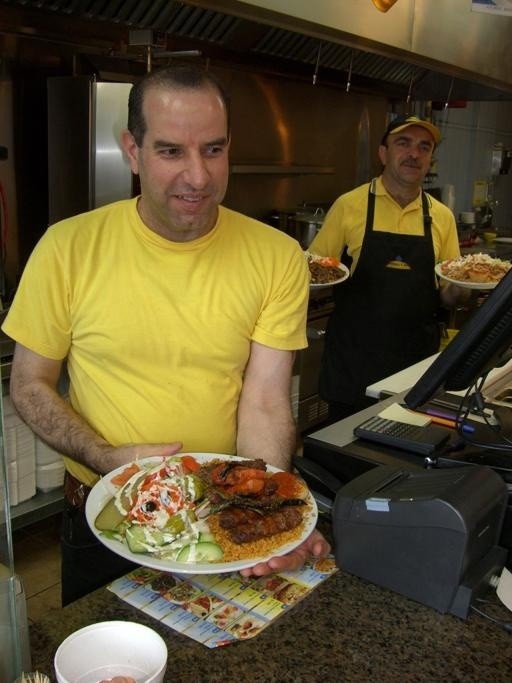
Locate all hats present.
[387,113,440,142]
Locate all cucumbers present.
[126,525,224,562]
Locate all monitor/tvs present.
[405,267,512,407]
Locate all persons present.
[9,64,332,610]
[310,114,492,420]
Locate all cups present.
[52,619,168,682]
[484,233,496,249]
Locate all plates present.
[434,262,501,290]
[86,453,317,575]
[493,237,512,243]
[460,211,475,224]
[309,256,349,291]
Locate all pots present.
[268,201,328,250]
